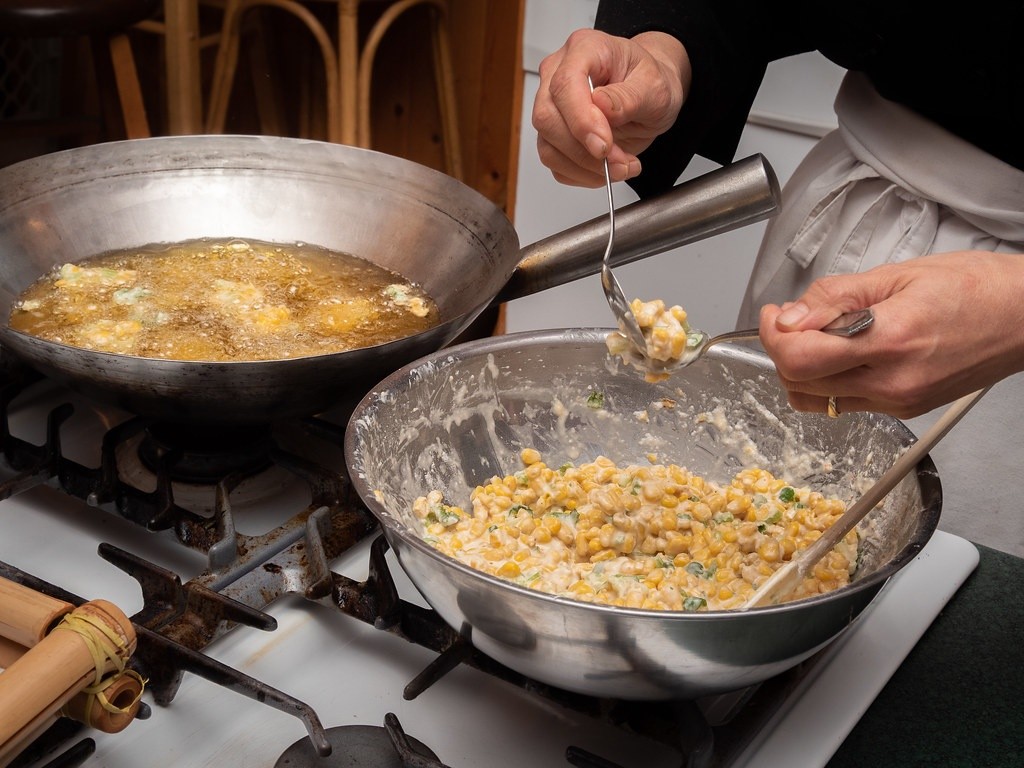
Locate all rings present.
[828,396,838,418]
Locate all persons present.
[531,0,1024,560]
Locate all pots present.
[0,143,781,400]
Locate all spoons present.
[588,77,648,359]
[621,307,872,369]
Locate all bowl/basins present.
[342,324,943,698]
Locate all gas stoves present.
[0,355,979,765]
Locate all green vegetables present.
[428,388,795,612]
